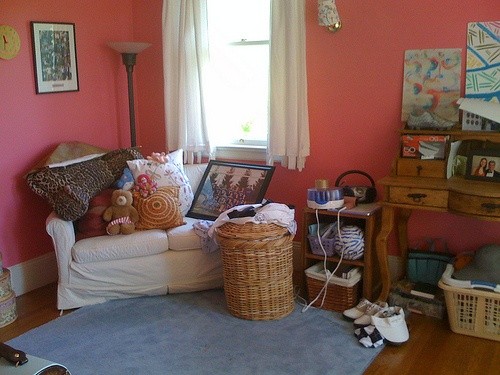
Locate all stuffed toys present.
[104,152,169,235]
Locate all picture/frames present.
[465,150,500,183]
[186,160,275,223]
[31,20,80,94]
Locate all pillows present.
[128,149,195,217]
[130,186,187,230]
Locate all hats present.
[452,244,500,283]
[371,306,410,343]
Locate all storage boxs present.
[389,278,445,321]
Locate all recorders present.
[343,186,377,204]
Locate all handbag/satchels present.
[0,340,71,375]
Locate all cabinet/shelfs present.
[300,201,382,302]
[376,123,500,305]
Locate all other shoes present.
[354,325,405,347]
[405,111,460,130]
[342,298,388,327]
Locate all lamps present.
[317,0,341,32]
[105,42,150,147]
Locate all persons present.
[203,181,248,213]
[472,158,500,178]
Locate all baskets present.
[215,221,296,321]
[437,257,500,343]
[304,260,362,312]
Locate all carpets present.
[3,287,385,375]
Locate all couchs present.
[45,163,226,311]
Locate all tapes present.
[316,179,329,189]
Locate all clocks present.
[0,24,21,61]
